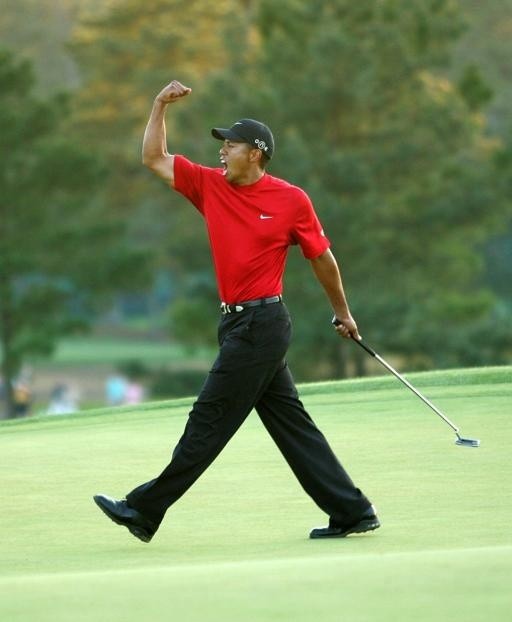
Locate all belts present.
[220,294,283,315]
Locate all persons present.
[93,80,381,543]
[8,359,144,415]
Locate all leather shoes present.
[311,505,379,538]
[93,493,158,542]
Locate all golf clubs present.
[333,317,481,447]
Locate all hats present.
[211,119,274,159]
